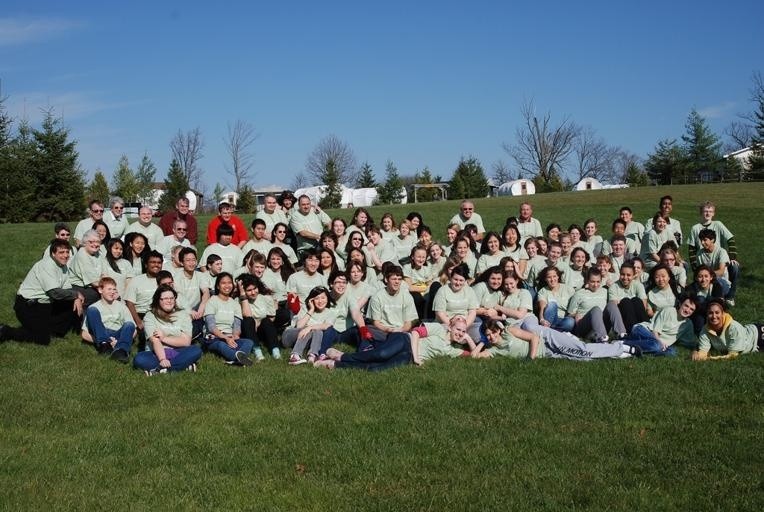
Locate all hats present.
[276,190,298,206]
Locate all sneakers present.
[271,346,281,361]
[326,347,344,361]
[235,350,253,368]
[144,367,161,377]
[0,322,11,345]
[111,349,131,361]
[598,332,641,359]
[253,347,265,363]
[314,359,336,369]
[184,362,197,372]
[318,352,328,361]
[99,342,110,353]
[287,353,308,366]
[306,352,317,364]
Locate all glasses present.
[159,297,176,302]
[334,281,348,286]
[277,230,287,233]
[174,227,187,231]
[113,206,124,210]
[461,207,474,211]
[91,209,104,213]
[353,237,363,242]
[59,233,71,237]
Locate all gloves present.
[359,326,377,349]
